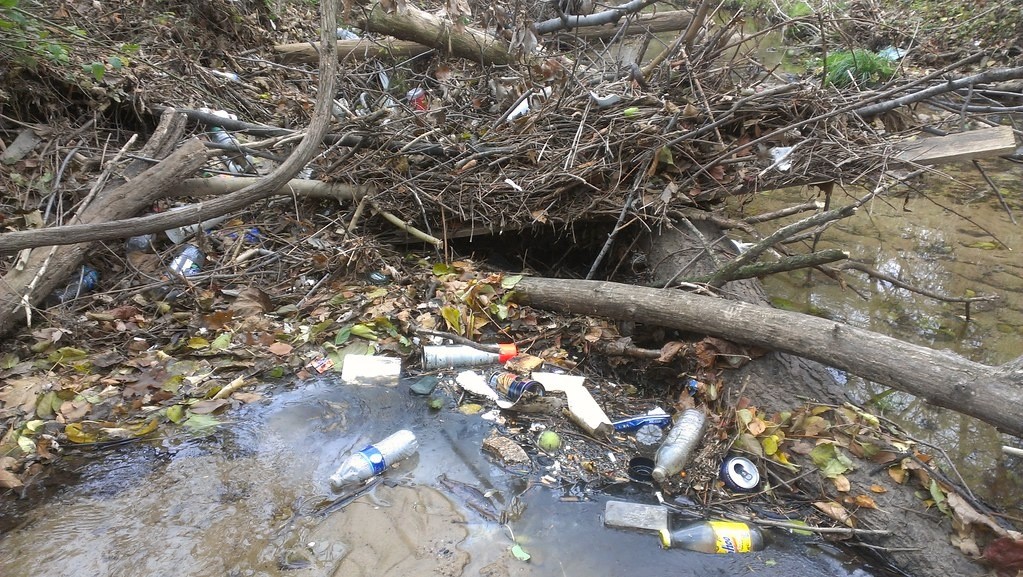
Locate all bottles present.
[207,228,260,243]
[164,244,205,276]
[224,73,238,81]
[422,344,517,370]
[368,271,389,287]
[661,520,763,554]
[212,127,252,173]
[337,28,360,40]
[123,208,159,251]
[330,430,419,487]
[652,408,706,483]
[406,88,429,111]
[54,267,99,303]
